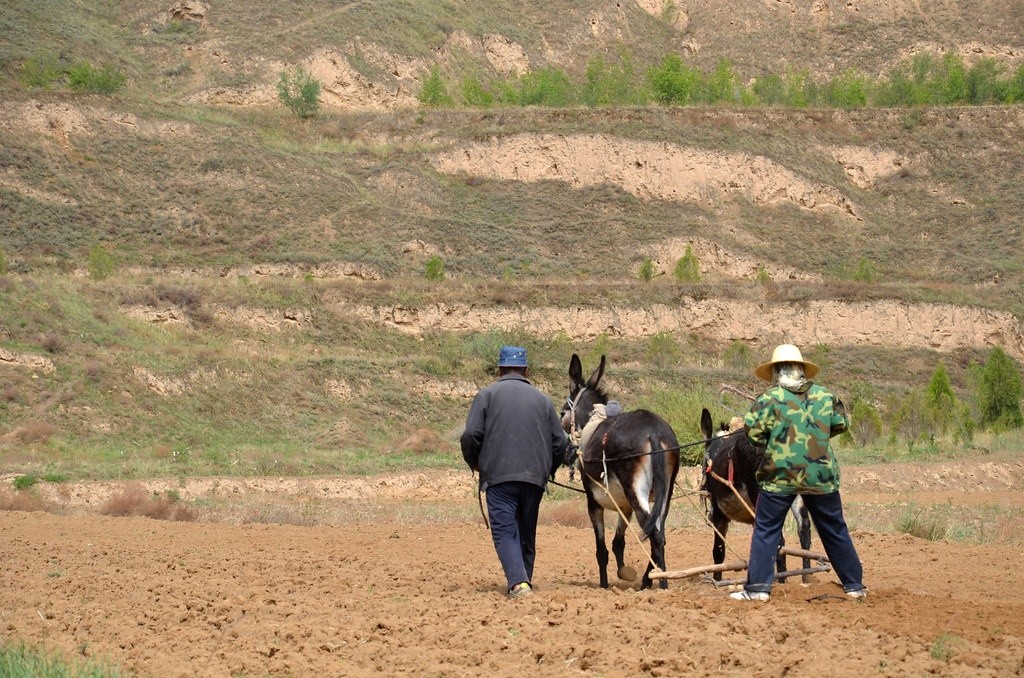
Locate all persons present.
[459,346,567,601]
[730,343,867,600]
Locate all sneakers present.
[846,589,869,602]
[506,583,533,602]
[729,591,770,603]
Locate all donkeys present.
[559,350,824,595]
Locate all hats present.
[497,346,528,367]
[756,344,820,384]
